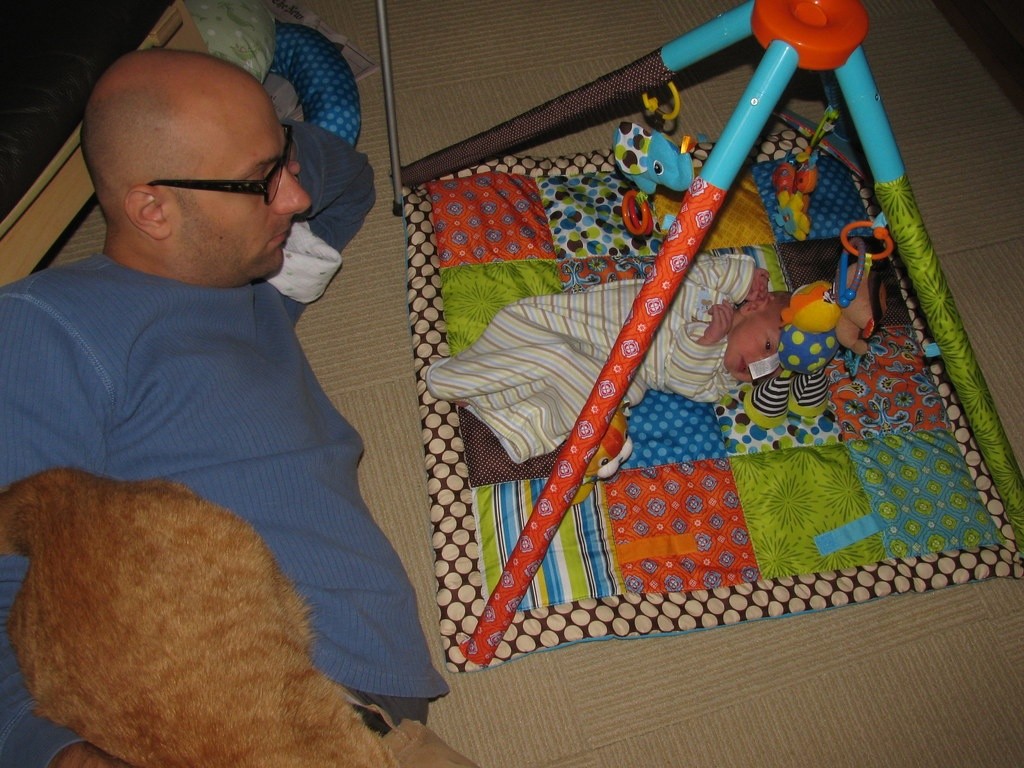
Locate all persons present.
[424,254,795,464]
[1,46,448,768]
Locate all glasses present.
[146,123,293,204]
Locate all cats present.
[0,466,402,767]
